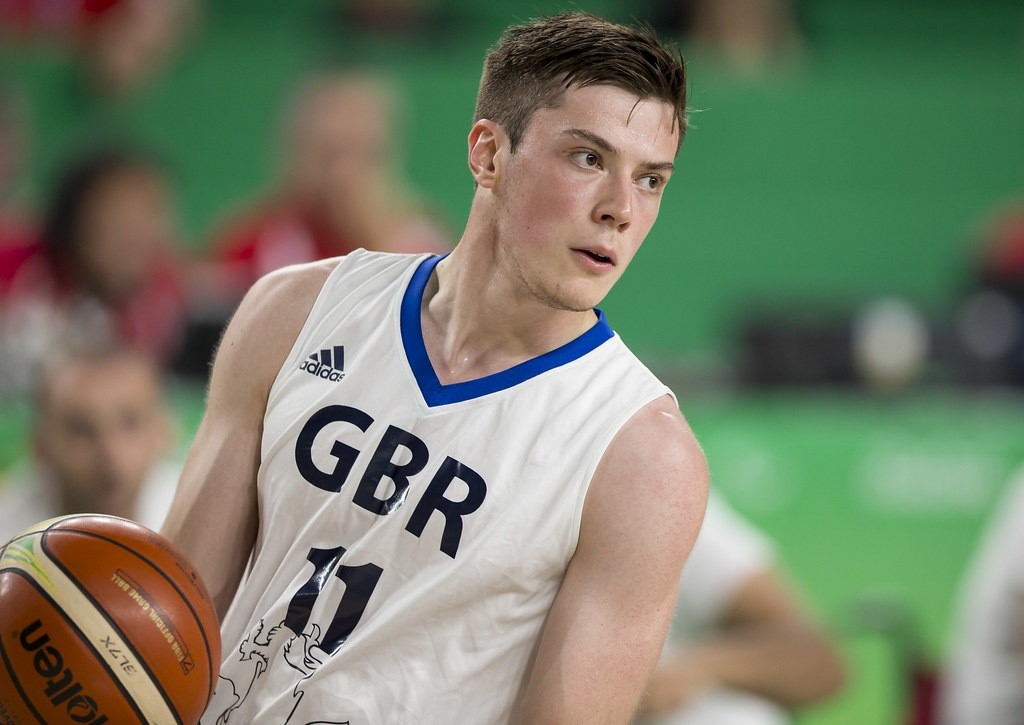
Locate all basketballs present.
[0,513,223,725]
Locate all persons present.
[0,0,850,725]
